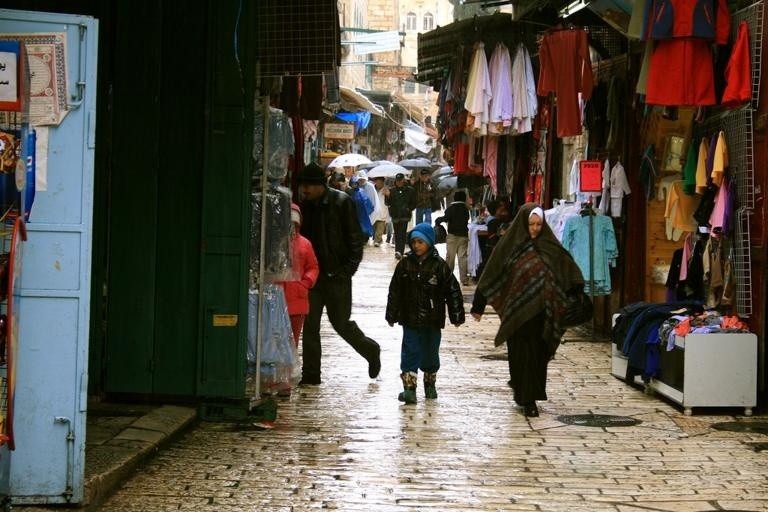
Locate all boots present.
[399,372,416,403]
[424,373,437,399]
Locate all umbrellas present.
[327,153,458,188]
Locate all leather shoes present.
[524,400,539,416]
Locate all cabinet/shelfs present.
[612,312,758,416]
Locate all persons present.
[485,200,502,259]
[328,170,444,259]
[471,203,585,417]
[260,203,319,401]
[386,223,465,404]
[436,190,469,286]
[298,161,381,384]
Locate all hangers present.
[689,121,726,144]
[583,203,596,216]
[613,156,624,169]
[266,181,280,194]
[249,276,268,291]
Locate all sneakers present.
[300,374,320,385]
[369,344,380,377]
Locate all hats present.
[410,230,432,247]
[291,203,301,224]
[298,163,327,184]
